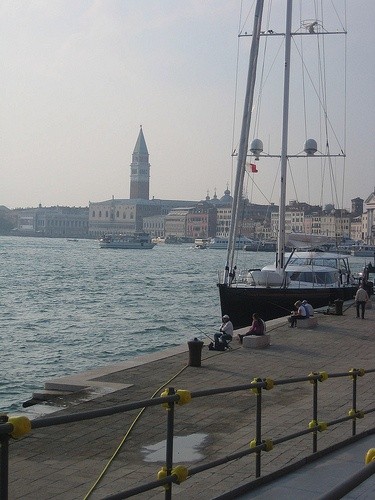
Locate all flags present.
[245,161,256,172]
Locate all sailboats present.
[216,0,375,330]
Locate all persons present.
[237,313,267,345]
[214,315,233,346]
[355,285,369,319]
[288,300,314,328]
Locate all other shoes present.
[238,333,241,339]
[289,325,293,328]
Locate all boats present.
[98,232,156,249]
[151,234,375,257]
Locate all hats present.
[302,300,307,303]
[223,315,229,319]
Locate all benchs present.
[242,335,271,348]
[297,316,318,327]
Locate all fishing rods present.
[186,318,216,342]
[271,302,293,313]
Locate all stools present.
[220,338,233,350]
